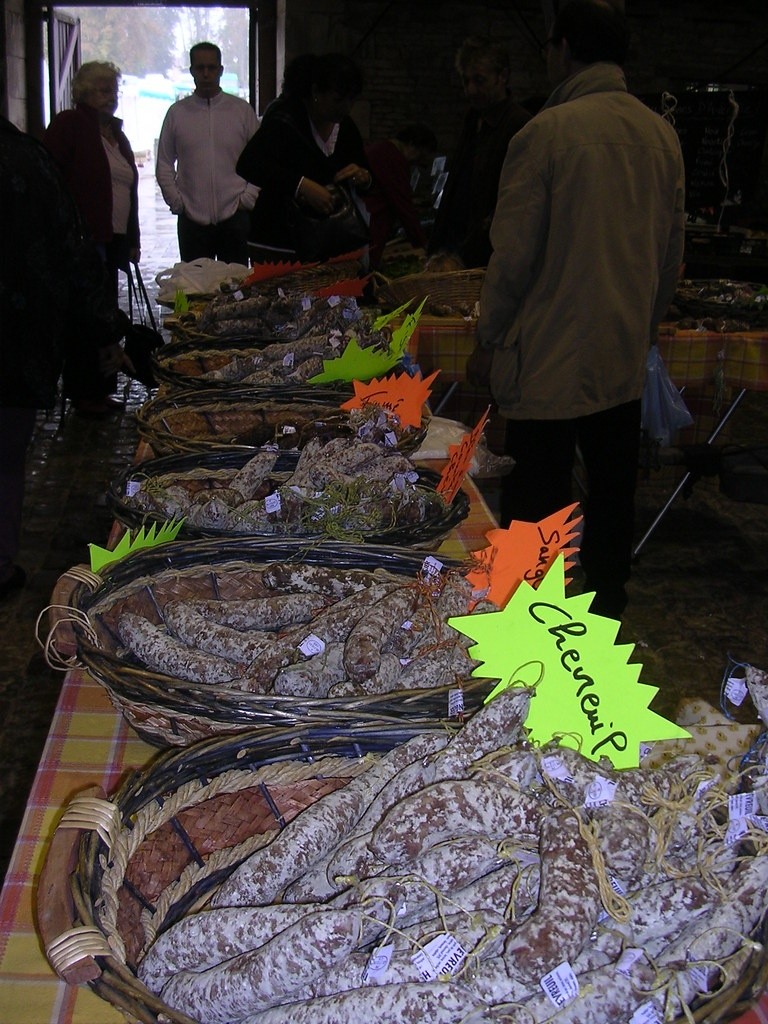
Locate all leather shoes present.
[60,390,126,417]
[0,564,26,600]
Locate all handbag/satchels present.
[293,182,370,252]
[121,324,164,389]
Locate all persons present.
[465,0,686,620]
[426,44,534,271]
[0,62,140,603]
[235,52,375,307]
[156,42,262,265]
[358,122,437,269]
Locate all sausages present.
[117,563,499,699]
[132,685,768,1024]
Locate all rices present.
[136,295,431,533]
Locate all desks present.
[0,389,760,1024]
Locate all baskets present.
[40,260,767,1024]
[371,268,487,313]
